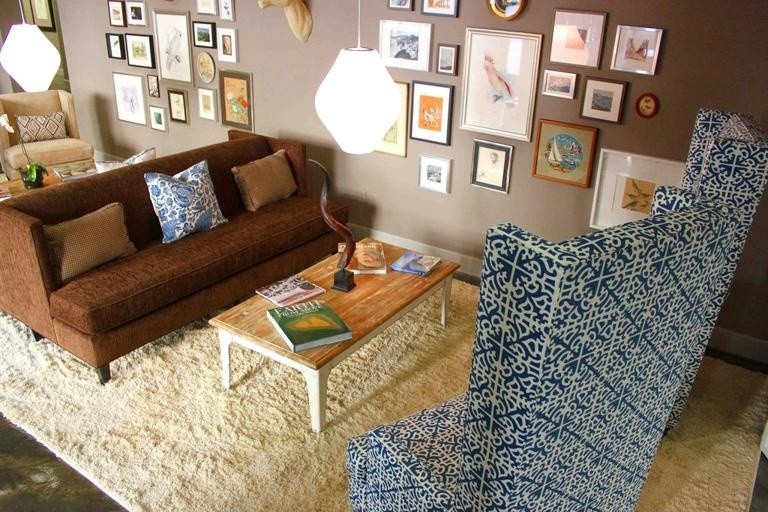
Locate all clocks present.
[635,92,660,117]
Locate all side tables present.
[1,175,65,199]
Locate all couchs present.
[2,90,97,181]
[1,131,351,386]
[346,107,767,512]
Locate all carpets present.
[2,278,765,511]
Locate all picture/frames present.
[457,26,544,143]
[374,81,410,158]
[484,0,528,22]
[419,0,460,18]
[378,19,433,73]
[530,118,601,189]
[107,0,127,27]
[218,0,235,21]
[196,0,217,16]
[610,24,665,76]
[469,138,515,195]
[216,27,239,63]
[218,68,254,132]
[147,74,160,98]
[197,87,218,121]
[167,89,190,125]
[546,7,611,71]
[191,20,217,48]
[589,147,686,232]
[124,33,156,69]
[151,9,194,87]
[29,1,57,33]
[541,69,580,100]
[148,105,168,132]
[196,51,215,83]
[105,32,126,60]
[409,80,456,146]
[111,72,148,126]
[578,75,631,126]
[124,1,148,27]
[435,42,461,76]
[417,154,454,194]
[387,0,415,11]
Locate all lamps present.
[314,46,404,155]
[1,21,62,93]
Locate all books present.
[256,275,325,307]
[0,186,12,201]
[336,242,387,274]
[267,300,352,351]
[53,162,95,181]
[390,252,441,277]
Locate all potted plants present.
[1,116,51,189]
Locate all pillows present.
[35,203,137,285]
[232,149,298,212]
[15,112,66,142]
[140,160,229,244]
